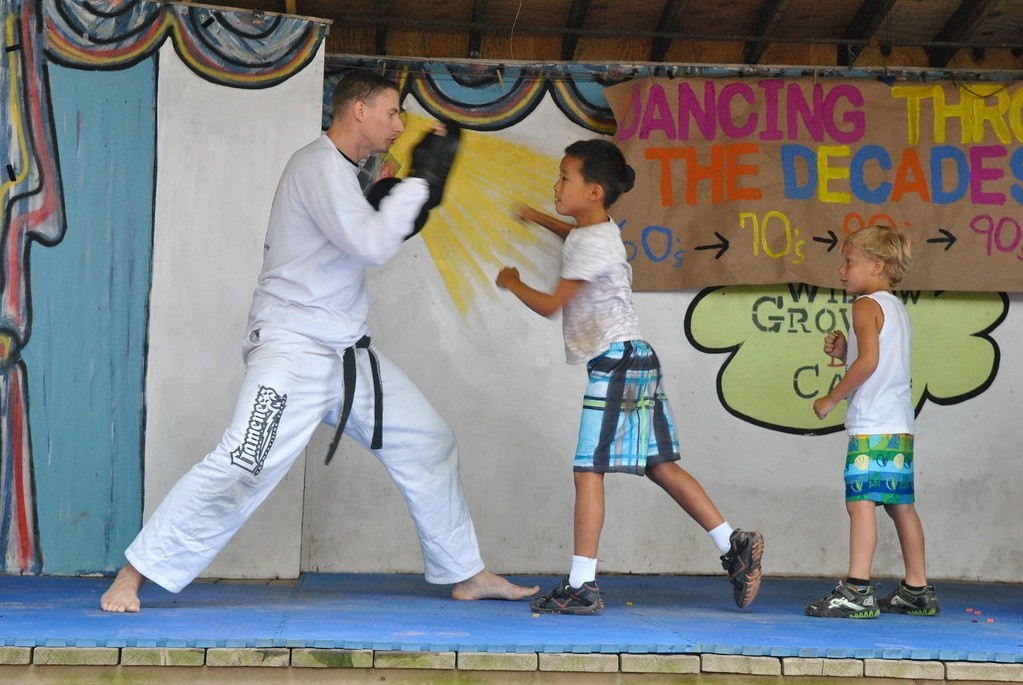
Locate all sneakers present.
[876,579,939,615]
[530,574,605,616]
[720,527,764,609]
[804,580,880,619]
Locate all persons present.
[100,69,541,613]
[495,139,765,616]
[804,224,941,620]
